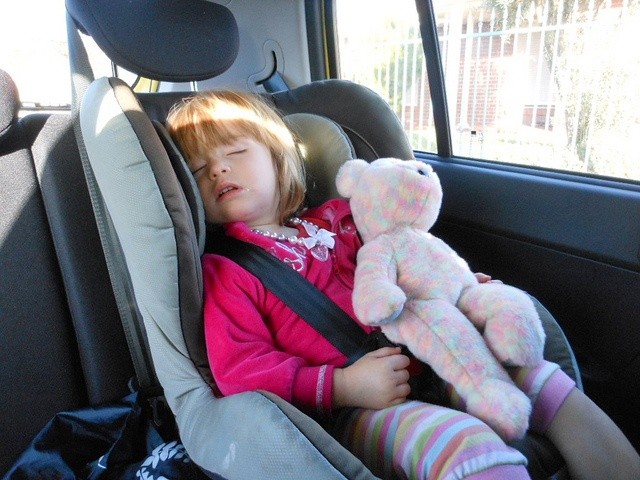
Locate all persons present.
[165,88,640,480]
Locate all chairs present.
[0,66,85,479]
[23,2,585,480]
